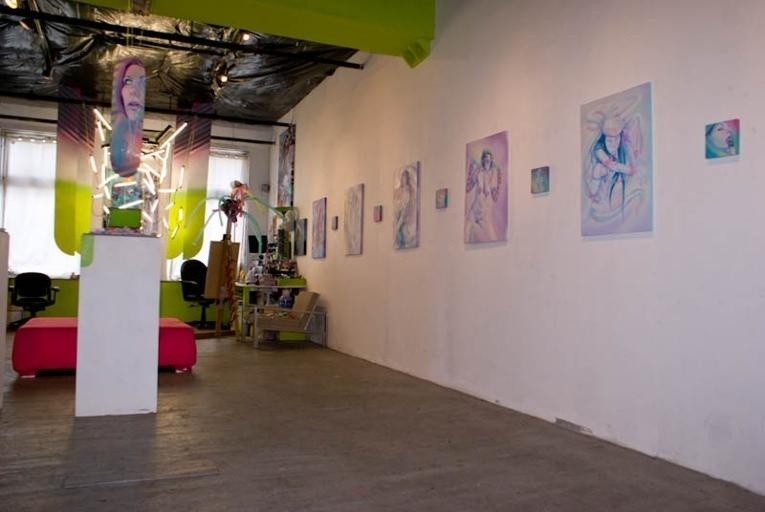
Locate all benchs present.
[12,316,195,382]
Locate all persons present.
[111,56,152,178]
[584,98,644,228]
[393,170,414,246]
[706,122,737,158]
[465,150,504,240]
[245,265,263,283]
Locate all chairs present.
[8,271,61,329]
[179,259,235,339]
[246,291,325,350]
